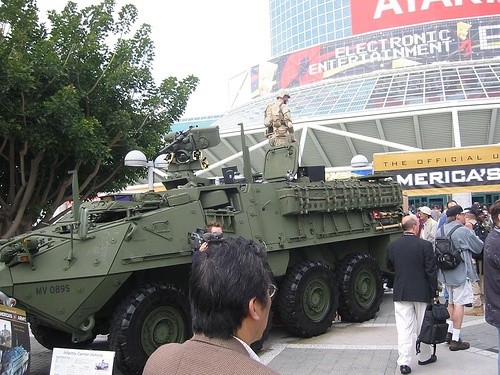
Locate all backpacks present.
[469,219,489,275]
[415,299,450,355]
[435,224,466,273]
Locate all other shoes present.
[443,299,448,307]
[465,303,472,307]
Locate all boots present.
[464,304,484,316]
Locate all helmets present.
[275,89,290,100]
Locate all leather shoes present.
[400,365,411,374]
[418,354,437,365]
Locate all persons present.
[142,237,281,375]
[386,198,500,375]
[264,89,296,146]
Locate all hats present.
[419,206,432,216]
[446,204,470,217]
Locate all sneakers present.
[446,332,461,344]
[449,338,470,351]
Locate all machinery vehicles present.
[0,122,406,375]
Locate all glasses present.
[268,283,278,297]
